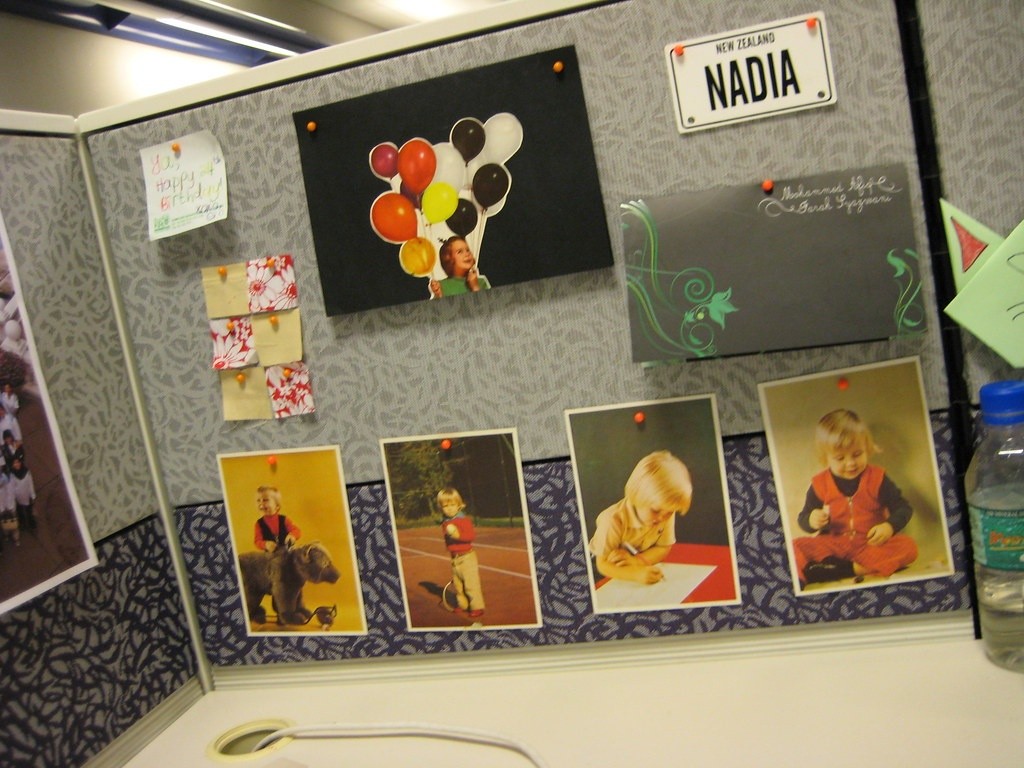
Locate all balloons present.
[370,111,525,278]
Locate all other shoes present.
[454,607,463,613]
[803,556,839,583]
[14,539,20,546]
[31,527,37,536]
[471,610,483,616]
[799,577,804,590]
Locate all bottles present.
[964,379,1024,674]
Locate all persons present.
[0,383,39,548]
[791,410,918,587]
[431,235,490,296]
[437,487,486,616]
[253,486,312,623]
[587,448,692,584]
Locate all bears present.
[235,540,341,624]
